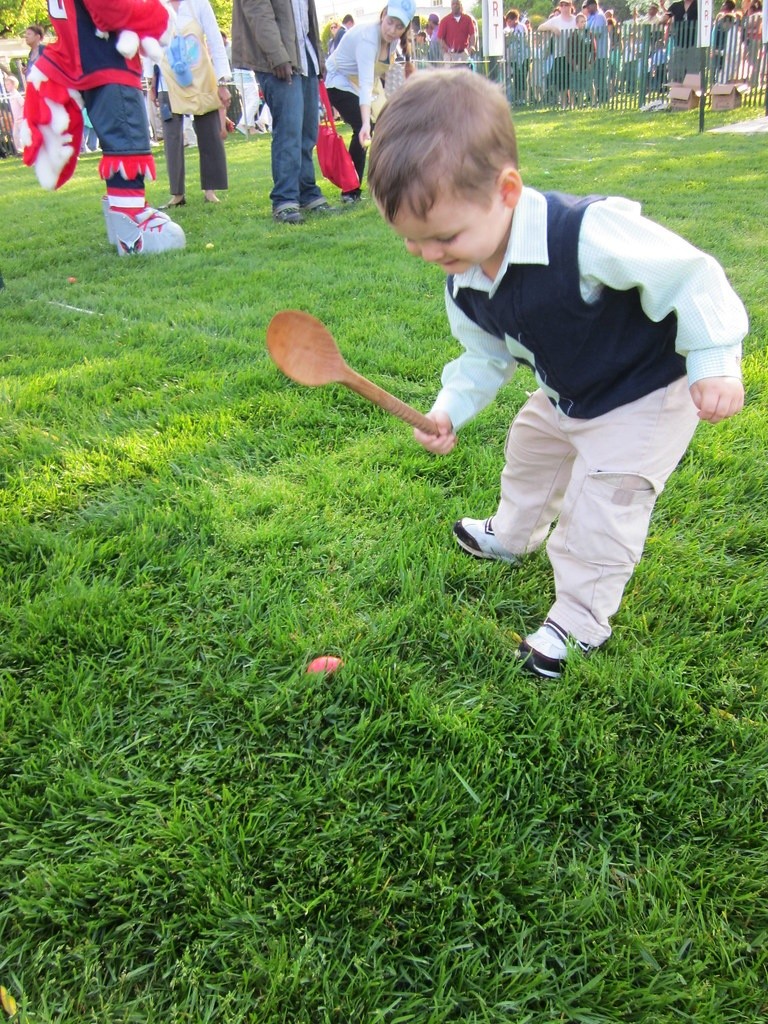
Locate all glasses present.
[330,26,337,29]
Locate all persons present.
[143,0,232,211]
[437,0,477,68]
[232,0,339,225]
[324,0,417,206]
[4,75,25,158]
[365,66,750,679]
[16,26,47,98]
[504,0,697,112]
[0,9,478,155]
[714,0,762,85]
[21,0,187,257]
[505,10,531,109]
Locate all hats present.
[582,0,596,9]
[387,0,416,28]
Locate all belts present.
[451,49,464,53]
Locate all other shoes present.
[343,194,361,204]
[278,207,305,224]
[205,195,221,204]
[315,202,340,215]
[156,196,186,210]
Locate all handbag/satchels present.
[316,83,361,193]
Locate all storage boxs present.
[706,82,750,112]
[662,73,702,111]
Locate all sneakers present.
[520,618,590,675]
[452,516,520,566]
